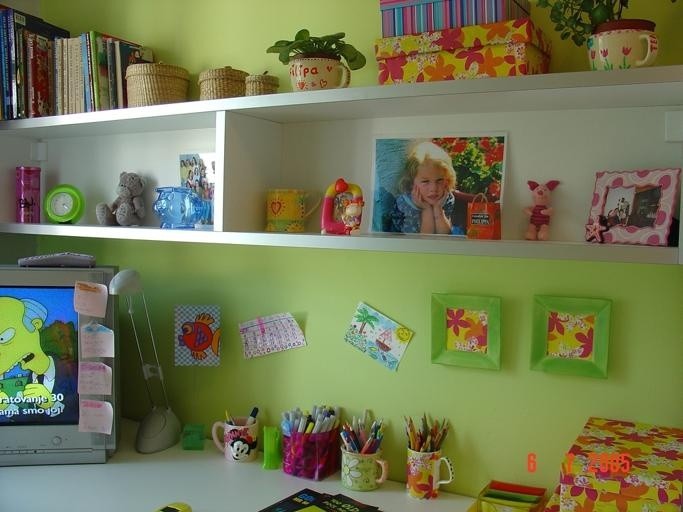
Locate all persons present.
[180,156,211,199]
[608,198,631,227]
[390,141,455,235]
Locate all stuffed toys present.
[523,177,560,242]
[96,171,146,227]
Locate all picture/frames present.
[429,292,504,372]
[530,295,612,379]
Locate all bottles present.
[11,164,39,224]
[148,187,205,231]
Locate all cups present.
[263,186,322,235]
[212,416,452,501]
[287,53,349,90]
[586,15,656,71]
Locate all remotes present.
[18,253,95,266]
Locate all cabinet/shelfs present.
[0,64,683,268]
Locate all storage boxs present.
[379,0,530,38]
[558,417,683,512]
[374,17,552,89]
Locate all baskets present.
[126,62,191,109]
[198,66,249,99]
[245,74,280,96]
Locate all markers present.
[245,407,259,425]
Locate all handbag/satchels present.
[468,194,500,239]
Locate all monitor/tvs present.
[0,264,120,466]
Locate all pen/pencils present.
[281,402,339,479]
[403,412,450,452]
[338,409,384,454]
[221,409,235,426]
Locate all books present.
[0,8,153,120]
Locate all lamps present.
[108,270,182,454]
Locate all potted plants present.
[264,28,367,92]
[536,0,659,70]
